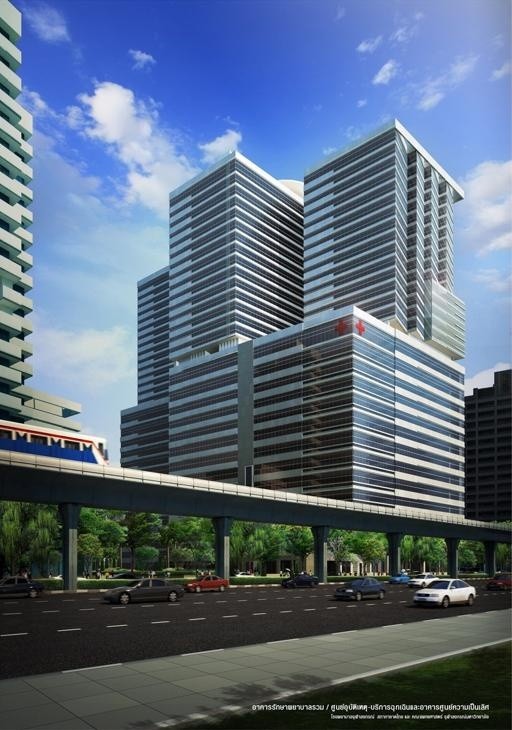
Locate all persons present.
[48,567,113,580]
[194,567,212,578]
[274,566,411,577]
[141,566,154,578]
